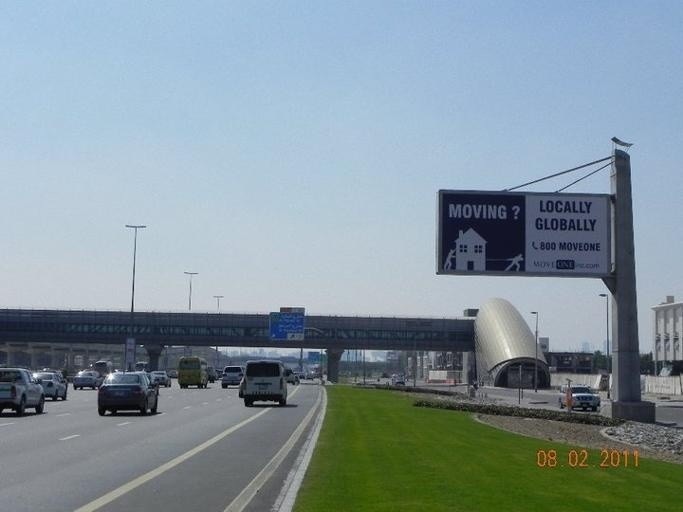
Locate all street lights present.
[212,295,224,313]
[597,291,610,373]
[123,224,146,312]
[183,271,198,311]
[530,311,539,393]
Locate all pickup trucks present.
[0,366,46,417]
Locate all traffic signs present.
[269,311,304,342]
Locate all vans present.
[236,359,289,408]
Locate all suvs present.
[390,372,406,386]
[558,380,601,413]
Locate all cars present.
[29,370,68,401]
[41,367,67,379]
[95,370,159,416]
[72,356,314,390]
